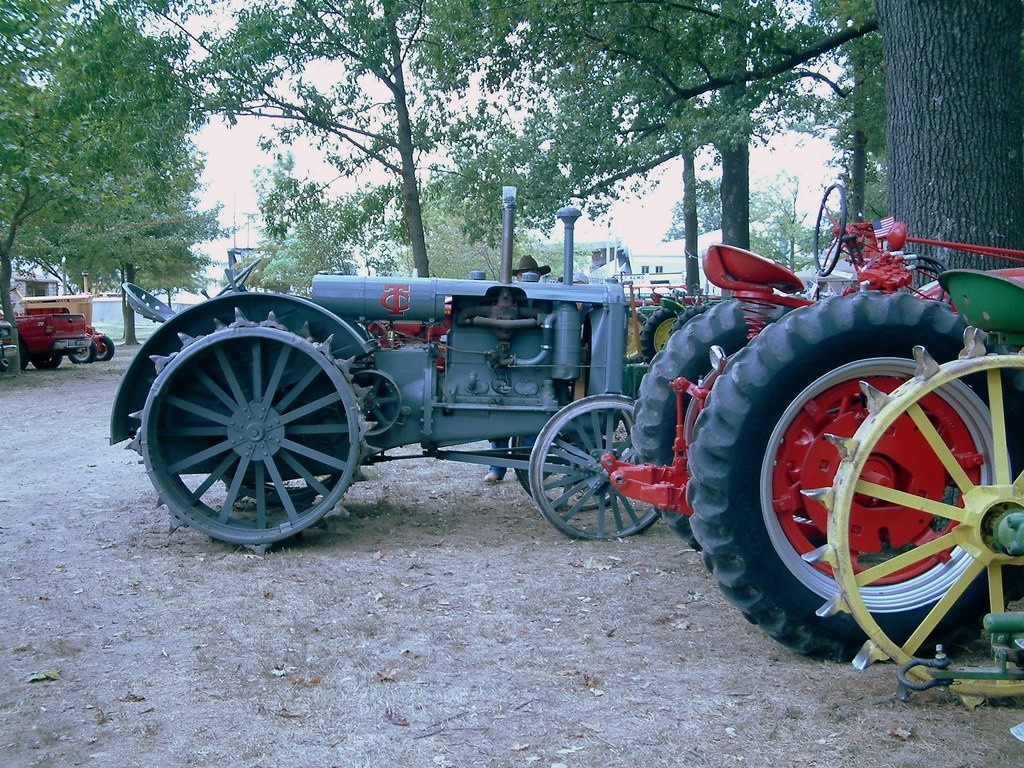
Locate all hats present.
[573,272,589,284]
[511,255,551,276]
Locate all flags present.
[872,217,895,238]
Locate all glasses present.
[518,269,540,276]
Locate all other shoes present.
[483,471,498,484]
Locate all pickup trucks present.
[0,307,98,371]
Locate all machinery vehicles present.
[106,184,678,557]
[600,181,1024,710]
[8,257,115,361]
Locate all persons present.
[482,255,552,482]
[557,273,591,324]
